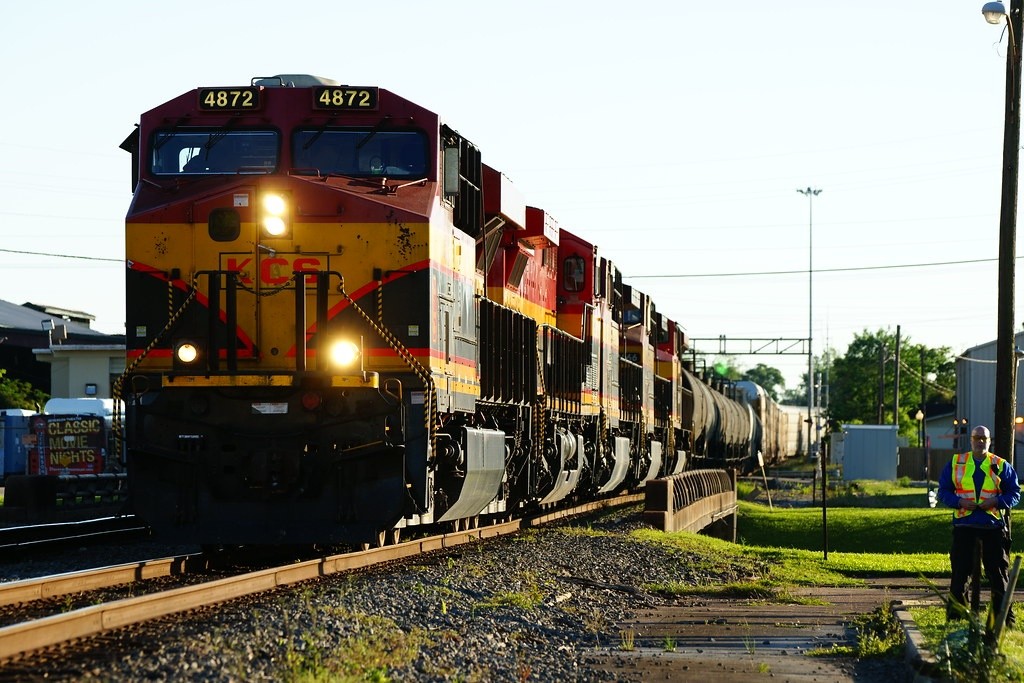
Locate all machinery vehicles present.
[0,398,125,506]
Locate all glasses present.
[971,435,991,440]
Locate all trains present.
[119,75,828,550]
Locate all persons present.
[937,426,1020,629]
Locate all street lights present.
[916,410,924,448]
[796,187,823,454]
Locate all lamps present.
[41,319,56,346]
[85,383,96,394]
